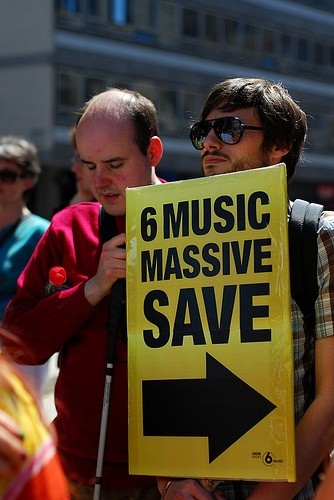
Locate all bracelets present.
[160,479,174,500]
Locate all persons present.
[0,83,173,500]
[0,137,53,326]
[0,348,74,500]
[55,110,98,214]
[153,77,334,500]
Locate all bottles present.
[45,265,70,295]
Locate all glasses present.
[0,168,33,183]
[190,115,265,150]
